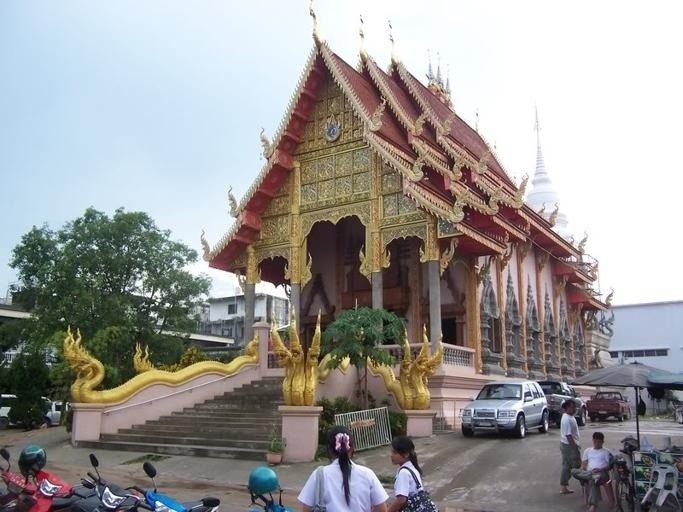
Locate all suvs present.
[457,381,549,438]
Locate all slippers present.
[587,506,596,511]
[594,475,600,488]
[559,487,575,494]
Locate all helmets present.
[18,445,47,476]
[249,468,282,495]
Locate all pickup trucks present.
[534,376,586,428]
[584,389,632,422]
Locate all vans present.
[0,393,50,430]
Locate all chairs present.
[638,463,682,512]
[581,476,614,508]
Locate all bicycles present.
[614,435,640,512]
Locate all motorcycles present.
[1,446,292,511]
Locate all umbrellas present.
[566,359,683,452]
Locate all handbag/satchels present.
[395,491,435,512]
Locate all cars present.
[35,398,73,426]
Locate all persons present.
[386,435,423,511]
[570,431,613,512]
[559,400,581,494]
[296,426,390,511]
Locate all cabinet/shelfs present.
[632,451,683,500]
